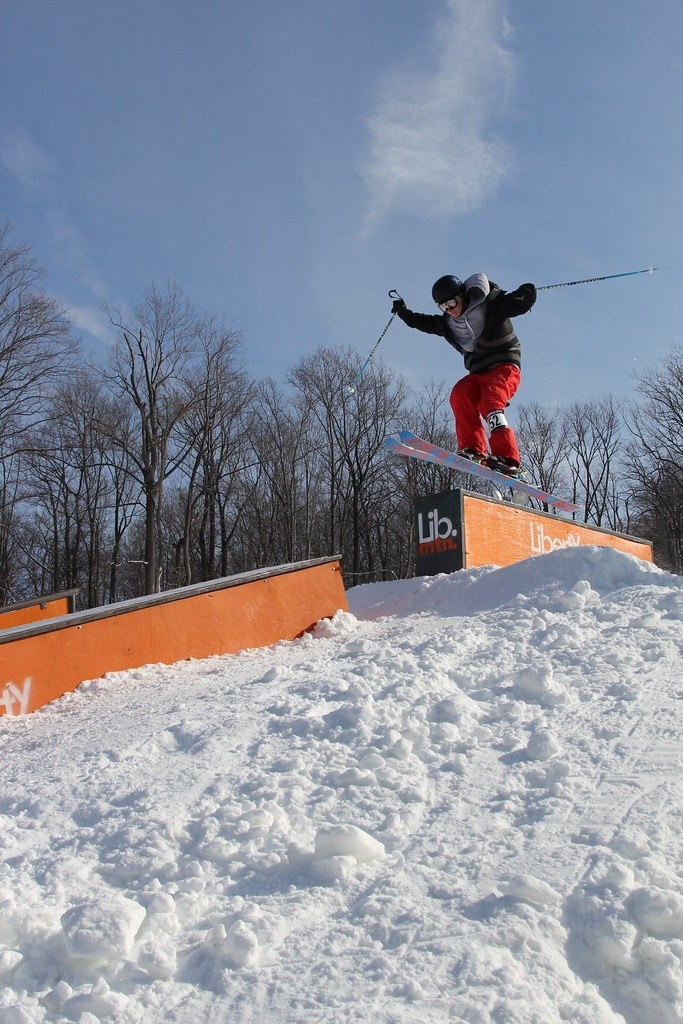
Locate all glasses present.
[438,293,462,312]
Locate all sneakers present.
[456,449,490,464]
[481,454,518,479]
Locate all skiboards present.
[384,430,583,514]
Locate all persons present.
[390,273,538,479]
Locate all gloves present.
[390,300,406,314]
[512,283,536,304]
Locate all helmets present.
[432,275,465,302]
